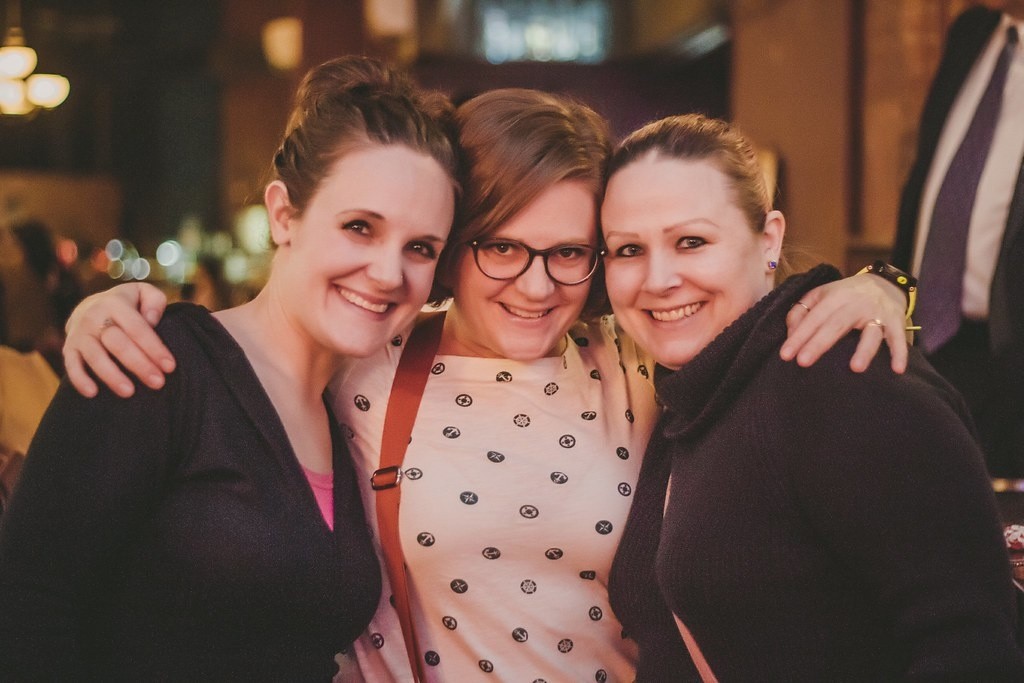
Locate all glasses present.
[466,237,606,286]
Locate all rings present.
[98,318,120,341]
[797,300,810,312]
[863,319,886,341]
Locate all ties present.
[911,25,1018,354]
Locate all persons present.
[0,55,1024,683]
[888,0,1024,478]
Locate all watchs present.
[854,256,923,330]
